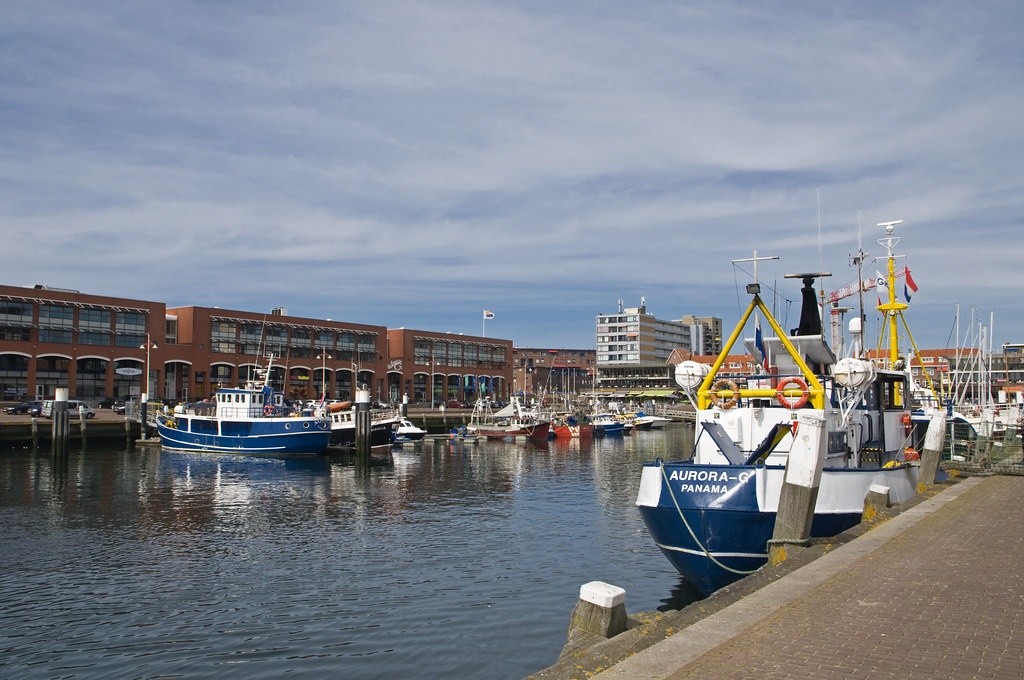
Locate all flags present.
[904,266,918,303]
[484,311,495,319]
[754,314,765,362]
[877,270,888,295]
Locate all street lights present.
[516,358,533,411]
[585,365,601,404]
[139,334,158,439]
[425,354,440,411]
[315,344,334,404]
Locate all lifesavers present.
[710,379,741,409]
[777,377,811,408]
[328,402,351,412]
[263,405,273,415]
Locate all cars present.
[160,399,179,408]
[370,400,391,409]
[2,400,43,416]
[26,402,43,417]
[444,399,509,409]
[97,394,141,413]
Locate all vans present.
[41,400,96,419]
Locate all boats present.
[392,407,428,441]
[158,312,334,456]
[298,399,401,447]
[465,216,1024,594]
